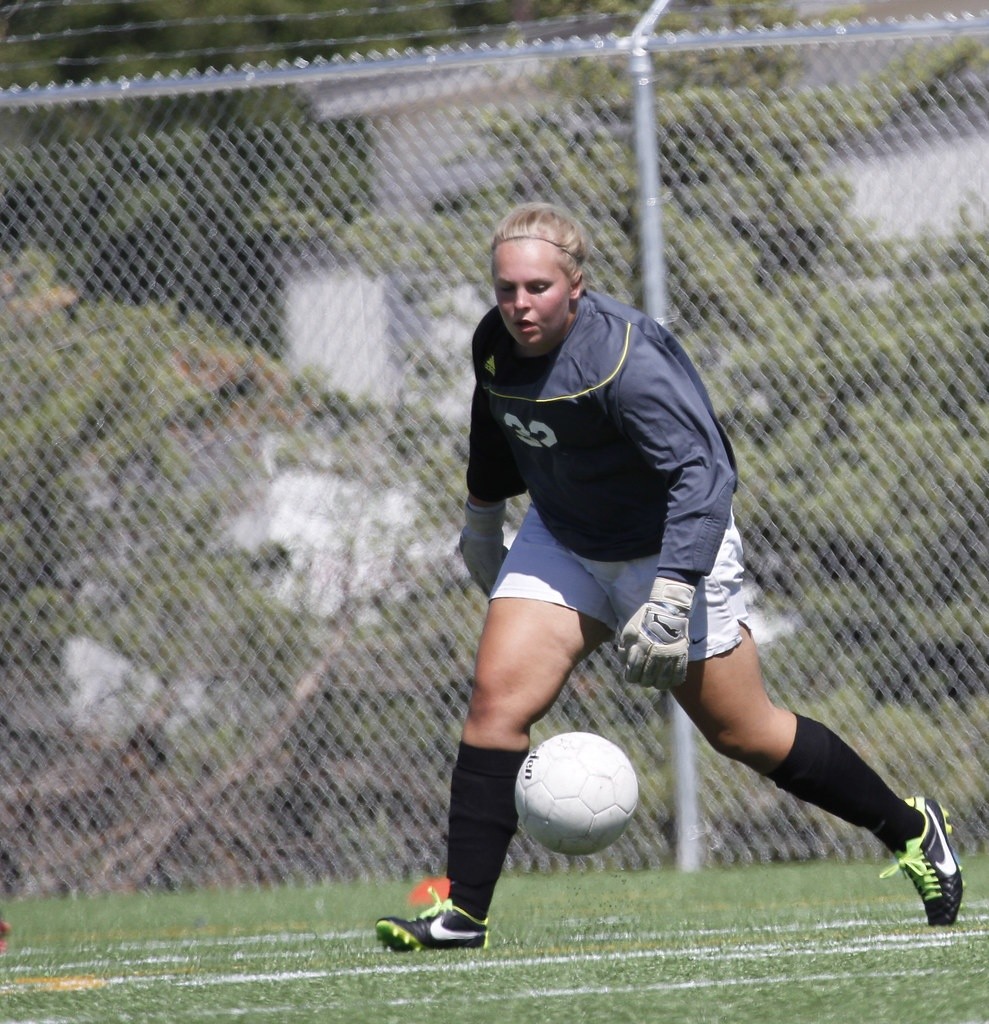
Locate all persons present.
[375,200,966,953]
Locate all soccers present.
[514,731,639,856]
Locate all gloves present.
[459,498,509,598]
[617,577,695,693]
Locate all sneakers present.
[375,886,491,953]
[878,796,967,926]
